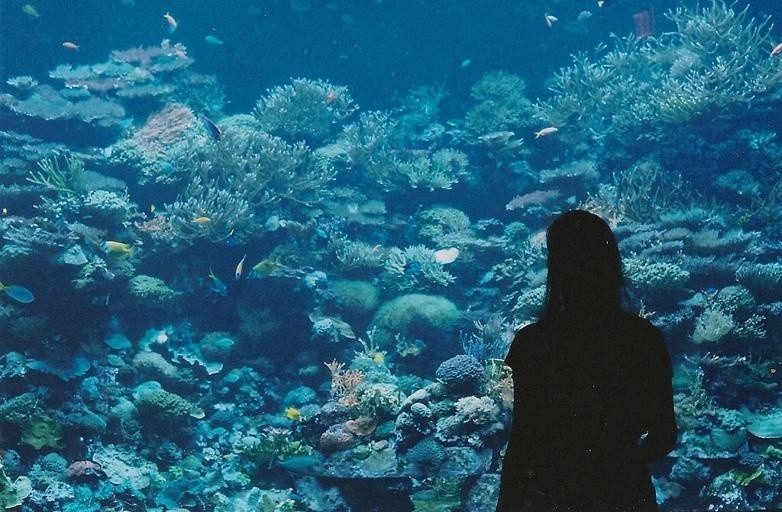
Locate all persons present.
[494,209,680,512]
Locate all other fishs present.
[0,0,782,509]
